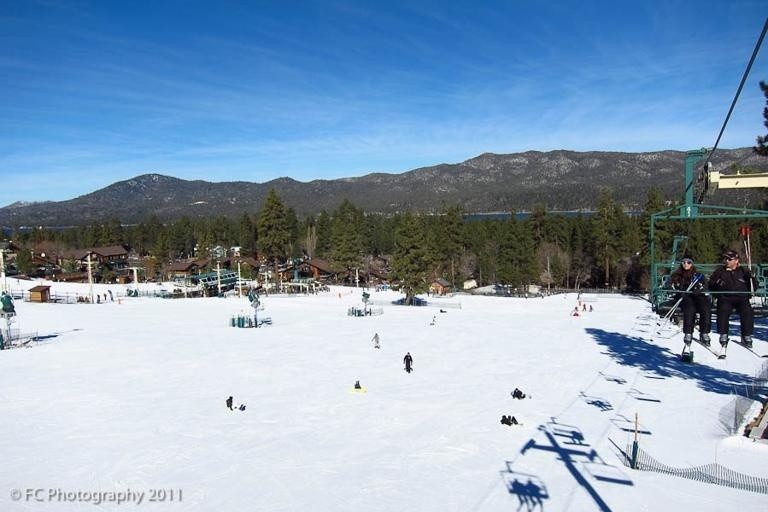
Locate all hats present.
[680,254,694,263]
[721,250,740,260]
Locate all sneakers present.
[719,333,729,345]
[700,333,711,343]
[741,335,753,346]
[684,333,692,345]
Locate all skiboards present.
[682,336,768,359]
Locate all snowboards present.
[511,392,532,399]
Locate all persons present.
[541,293,544,299]
[525,292,528,299]
[572,294,593,316]
[513,388,522,400]
[501,415,518,426]
[226,396,233,407]
[403,351,416,374]
[665,250,759,346]
[373,333,380,347]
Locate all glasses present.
[681,261,692,265]
[725,257,732,261]
